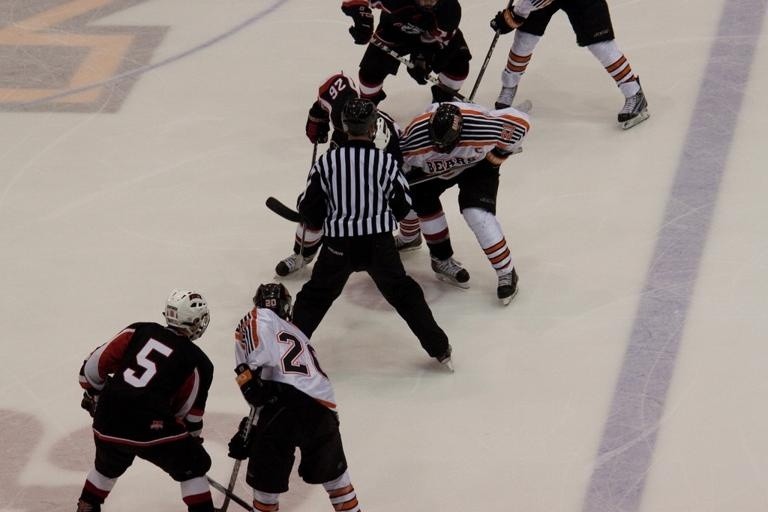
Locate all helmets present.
[161,290,211,341]
[341,96,379,135]
[252,282,293,324]
[427,103,464,155]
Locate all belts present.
[321,232,395,248]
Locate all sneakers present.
[430,257,470,283]
[614,79,648,123]
[275,250,315,277]
[494,67,524,111]
[389,230,423,251]
[494,265,519,299]
[435,344,452,365]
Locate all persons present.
[490,0,650,132]
[75,287,222,512]
[277,95,453,375]
[271,75,423,276]
[226,283,359,511]
[340,0,472,109]
[395,100,533,306]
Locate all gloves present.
[306,100,330,145]
[406,46,436,84]
[179,418,203,447]
[234,362,275,410]
[490,6,525,34]
[478,158,500,180]
[341,1,374,45]
[81,389,97,417]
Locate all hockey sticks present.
[467,0,514,102]
[290,141,319,273]
[220,405,256,512]
[368,34,532,115]
[265,147,524,223]
[207,475,254,511]
